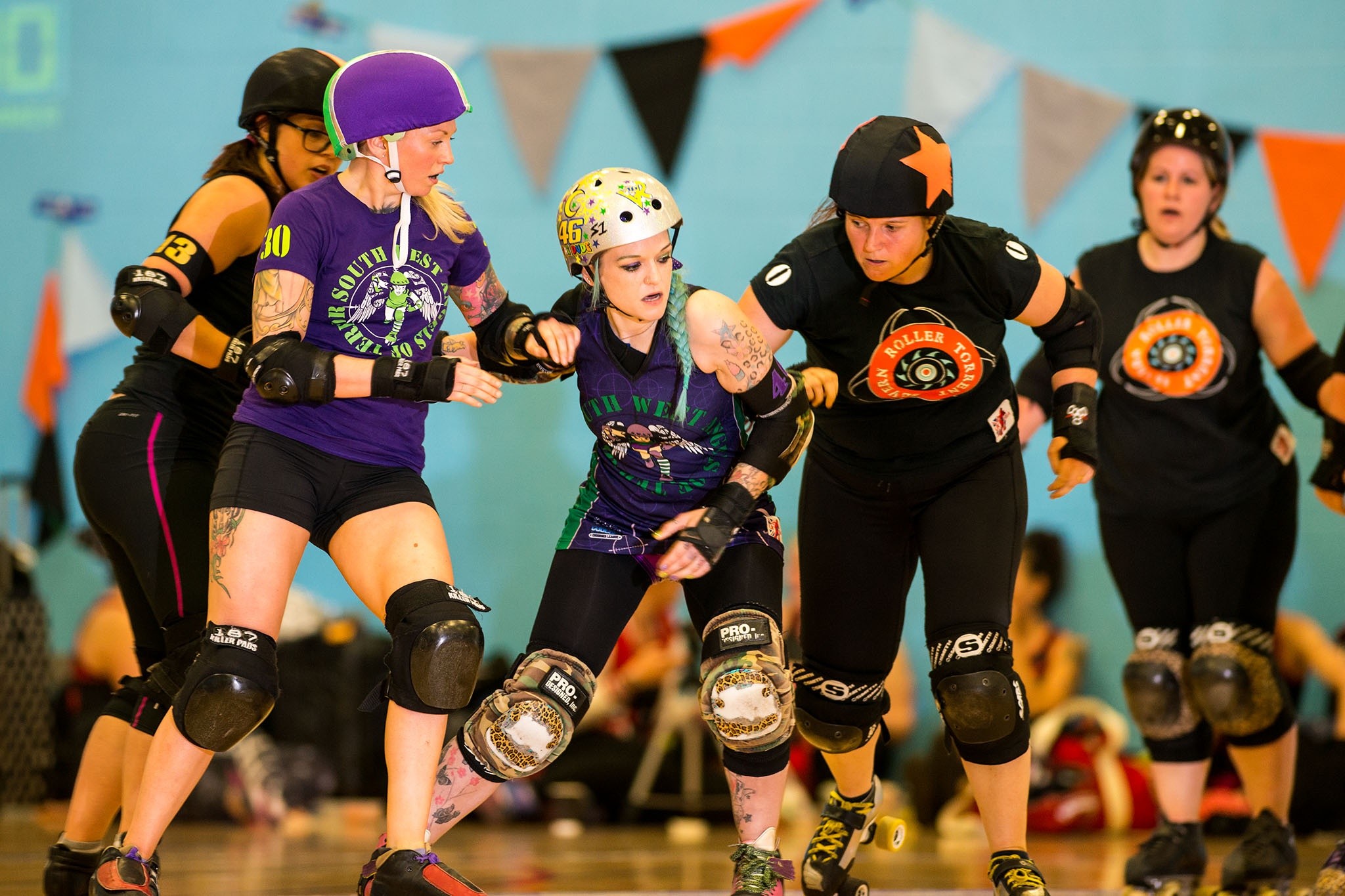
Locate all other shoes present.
[1313,838,1345,895]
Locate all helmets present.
[557,168,683,275]
[238,47,347,134]
[1129,109,1229,189]
[325,48,472,159]
[827,116,953,218]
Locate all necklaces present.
[618,320,656,341]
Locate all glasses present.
[265,116,330,153]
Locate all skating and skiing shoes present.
[1211,809,1312,896]
[1119,821,1206,896]
[987,848,1051,896]
[799,775,908,896]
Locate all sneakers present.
[91,845,159,896]
[43,831,107,896]
[356,831,388,896]
[364,848,488,896]
[729,843,795,896]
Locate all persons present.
[381,104,1344,896]
[78,50,582,896]
[42,48,348,896]
